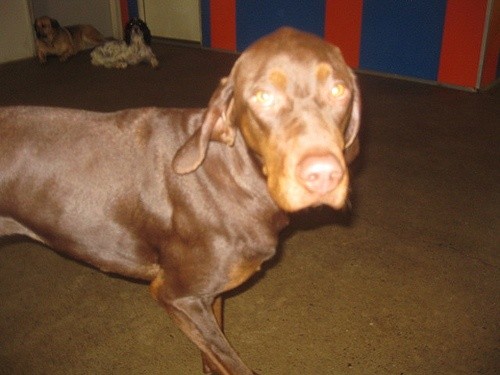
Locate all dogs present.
[90,19,159,69]
[33,16,114,62]
[0,26,363,374]
